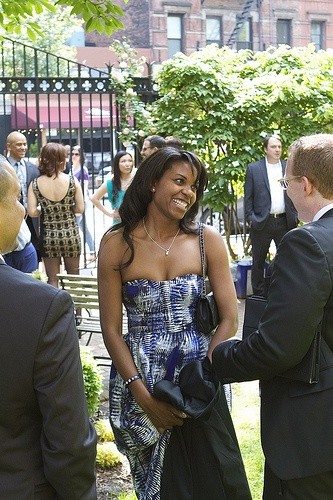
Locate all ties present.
[15,162,23,184]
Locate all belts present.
[267,212,286,219]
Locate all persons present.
[141,134,185,161]
[0,153,98,500]
[28,143,85,325]
[0,219,38,274]
[7,131,42,262]
[243,134,299,295]
[68,145,98,265]
[98,148,239,500]
[90,151,135,226]
[211,133,333,500]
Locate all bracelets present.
[126,374,142,385]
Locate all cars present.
[194,187,251,237]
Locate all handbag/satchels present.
[242,293,322,387]
[194,221,219,337]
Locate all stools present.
[236,260,268,298]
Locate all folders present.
[241,295,321,384]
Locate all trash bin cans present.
[235,260,269,299]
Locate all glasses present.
[71,153,79,155]
[141,146,154,151]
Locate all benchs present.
[56,275,130,367]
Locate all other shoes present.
[75,315,84,327]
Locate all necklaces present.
[143,216,181,255]
